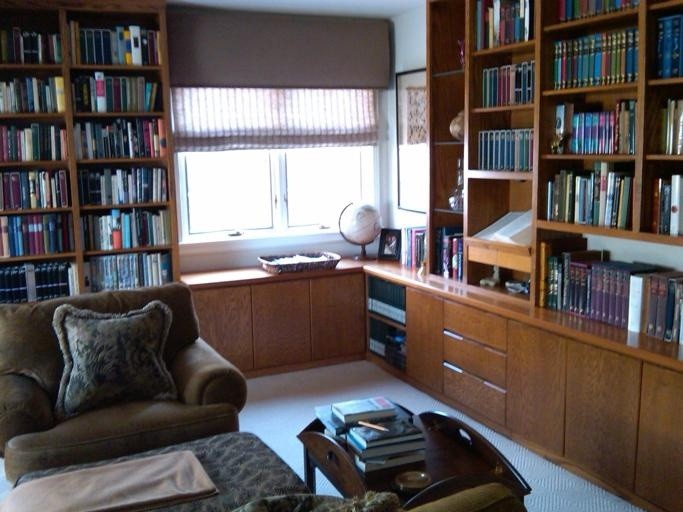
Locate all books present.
[404,226,427,270]
[541,0,640,231]
[78,27,172,294]
[436,224,463,282]
[644,15,683,239]
[312,395,428,474]
[0,21,77,304]
[537,233,682,348]
[465,0,535,172]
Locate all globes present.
[338,202,382,260]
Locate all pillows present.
[233,490,399,512]
[51,300,178,419]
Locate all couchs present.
[0,431,309,512]
[233,481,527,512]
[0,283,246,485]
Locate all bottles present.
[448,158,463,213]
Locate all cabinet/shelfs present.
[0,0,181,303]
[403,285,682,512]
[191,272,368,373]
[426,0,681,337]
[362,270,405,382]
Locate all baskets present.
[258,252,341,273]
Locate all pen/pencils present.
[358,421,389,431]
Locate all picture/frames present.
[394,67,431,214]
[377,228,401,262]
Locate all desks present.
[297,401,531,512]
